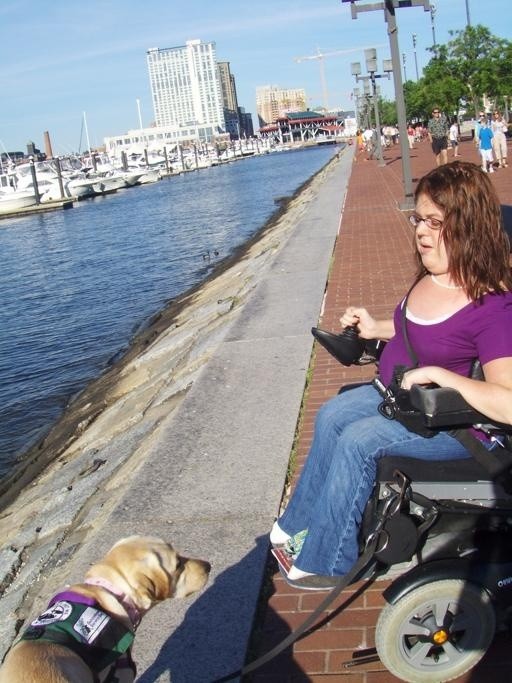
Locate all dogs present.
[0,535,211,683]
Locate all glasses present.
[409,216,443,229]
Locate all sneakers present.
[481,163,508,173]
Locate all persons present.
[334,144,341,162]
[348,107,510,174]
[268,160,512,592]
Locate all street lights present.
[350,47,394,168]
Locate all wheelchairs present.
[312,205,510,683]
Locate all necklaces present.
[430,272,466,289]
